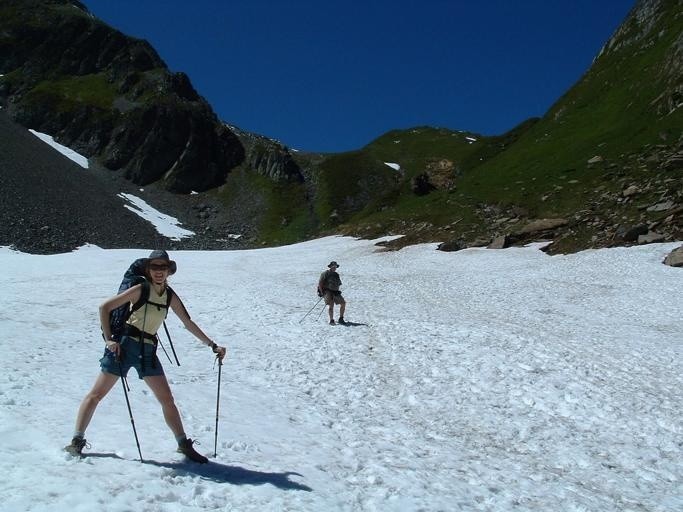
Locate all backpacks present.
[100,258,171,342]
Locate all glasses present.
[148,264,168,270]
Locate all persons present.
[64,248,227,464]
[318,261,347,326]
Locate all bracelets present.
[208,341,213,346]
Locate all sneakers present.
[330,320,335,326]
[339,317,346,325]
[65,438,87,459]
[177,438,209,463]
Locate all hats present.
[328,261,339,268]
[146,250,176,274]
